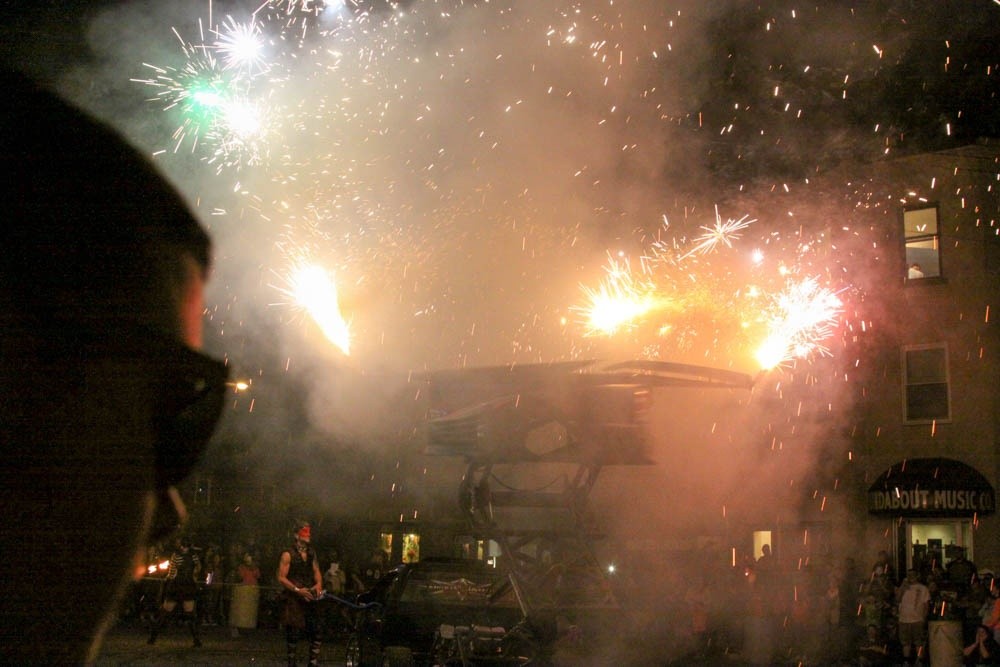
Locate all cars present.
[344,556,551,667]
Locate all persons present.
[683,542,1000,667]
[0,67,215,667]
[143,519,404,667]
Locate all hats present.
[294,524,310,543]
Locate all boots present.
[147,606,172,644]
[186,609,203,647]
